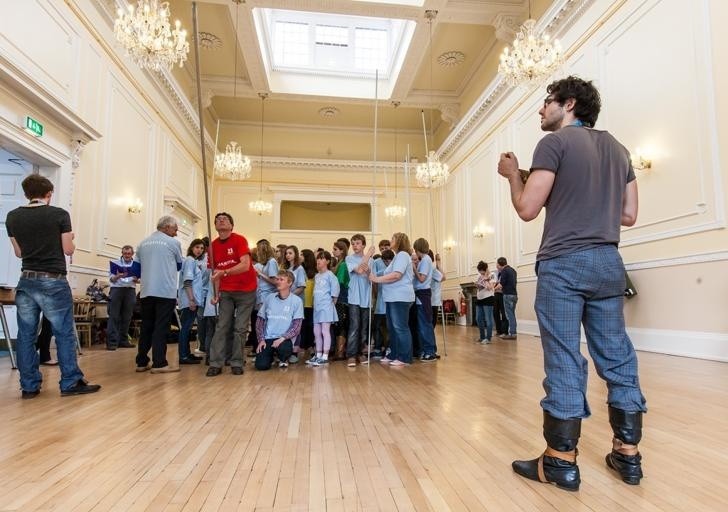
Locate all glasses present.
[544,99,556,106]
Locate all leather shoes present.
[44,360,58,366]
[512,449,581,491]
[606,441,643,485]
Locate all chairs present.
[442,300,457,326]
[436,301,442,325]
[73,295,93,350]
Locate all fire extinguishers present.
[460,296,468,314]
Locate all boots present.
[334,336,345,359]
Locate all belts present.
[22,271,58,278]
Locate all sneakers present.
[106,346,115,350]
[289,355,299,363]
[22,389,40,399]
[477,338,490,344]
[231,366,242,375]
[207,366,221,376]
[495,334,517,339]
[118,343,135,348]
[279,360,288,367]
[61,380,100,395]
[419,353,440,362]
[136,363,151,371]
[347,343,408,367]
[305,355,329,366]
[180,349,204,364]
[150,366,180,374]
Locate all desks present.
[0,286,18,369]
[73,300,110,349]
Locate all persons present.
[105,211,446,377]
[473,257,517,345]
[36,312,58,365]
[5,174,101,398]
[498,76,647,493]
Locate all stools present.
[130,319,142,346]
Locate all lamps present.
[629,129,673,173]
[247,93,274,216]
[110,0,190,73]
[212,0,251,183]
[414,10,452,189]
[498,0,565,91]
[384,100,408,218]
